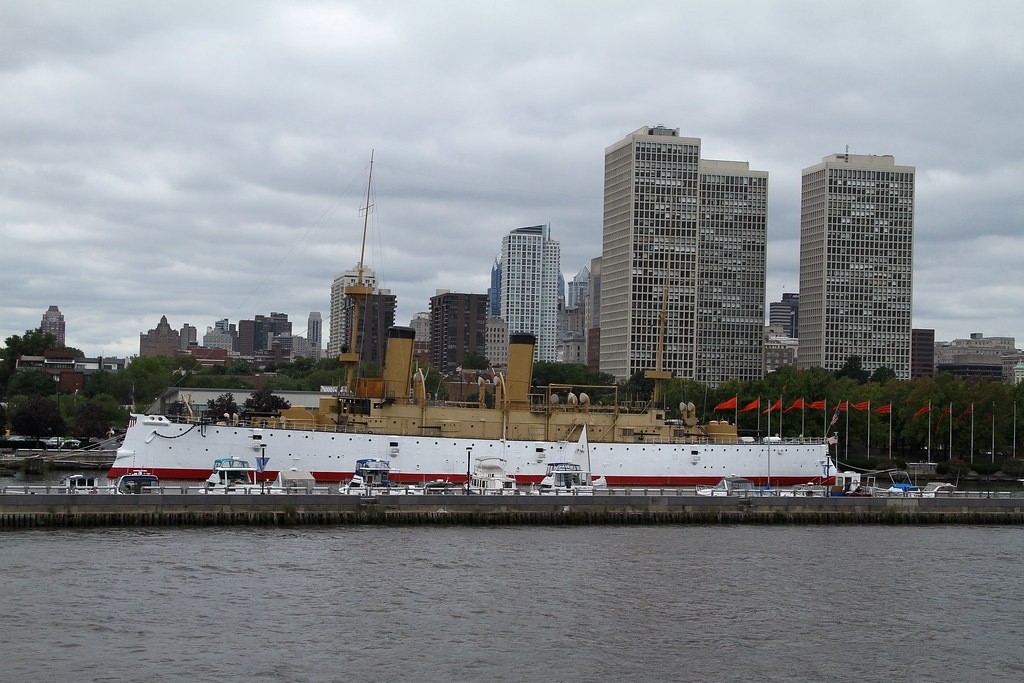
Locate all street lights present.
[465,445,473,495]
[259,444,266,493]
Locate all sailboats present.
[536,421,607,490]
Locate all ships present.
[108,146,845,492]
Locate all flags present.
[1008,404,1017,418]
[955,405,972,424]
[713,397,736,412]
[737,398,760,412]
[763,399,782,414]
[872,403,892,414]
[829,401,848,413]
[986,406,999,423]
[781,396,804,413]
[827,435,838,445]
[912,401,930,418]
[936,405,950,434]
[850,401,868,411]
[804,399,825,411]
[830,407,840,426]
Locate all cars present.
[8,435,78,448]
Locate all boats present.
[695,474,767,497]
[198,455,321,494]
[847,470,959,498]
[463,454,517,495]
[113,467,163,495]
[57,475,100,495]
[337,457,414,494]
